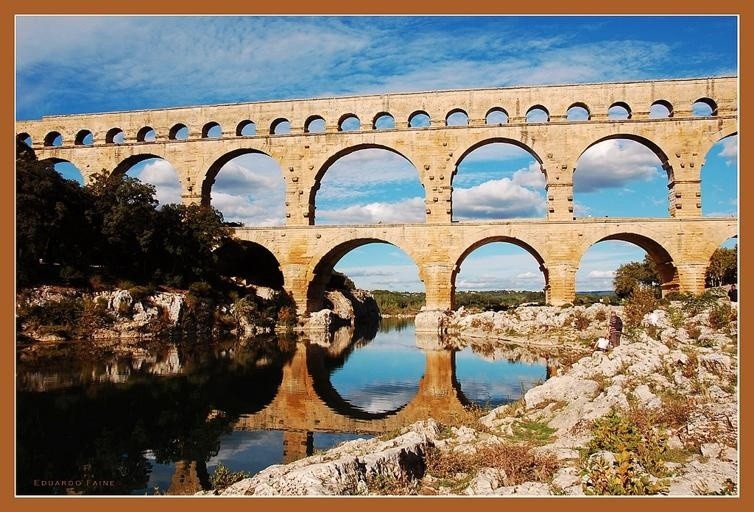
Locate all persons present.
[607,309,623,348]
[592,332,614,353]
[727,284,737,311]
